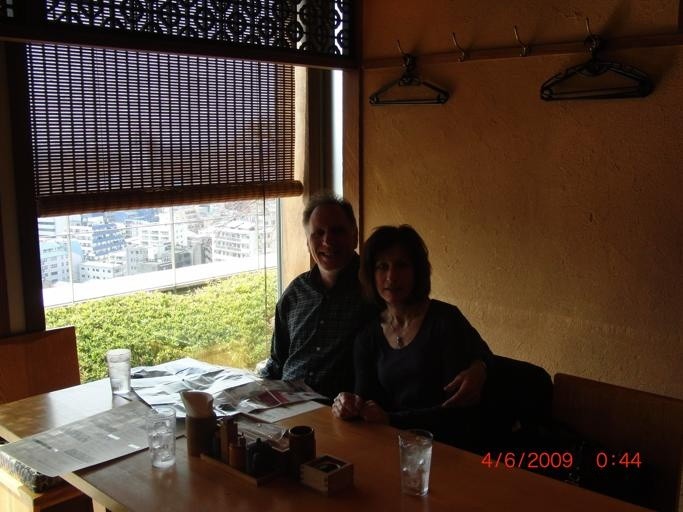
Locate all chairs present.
[481,353,557,478]
[1,324,86,510]
[551,370,682,511]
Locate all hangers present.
[367,52,450,106]
[538,35,656,101]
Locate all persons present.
[259,195,496,409]
[331,224,475,445]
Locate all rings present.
[457,390,467,400]
[364,401,368,406]
[450,381,459,388]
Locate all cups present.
[289,425,317,465]
[104,348,135,396]
[398,428,432,498]
[185,412,214,458]
[144,407,177,470]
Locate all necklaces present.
[386,317,414,345]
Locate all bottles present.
[229,436,270,478]
